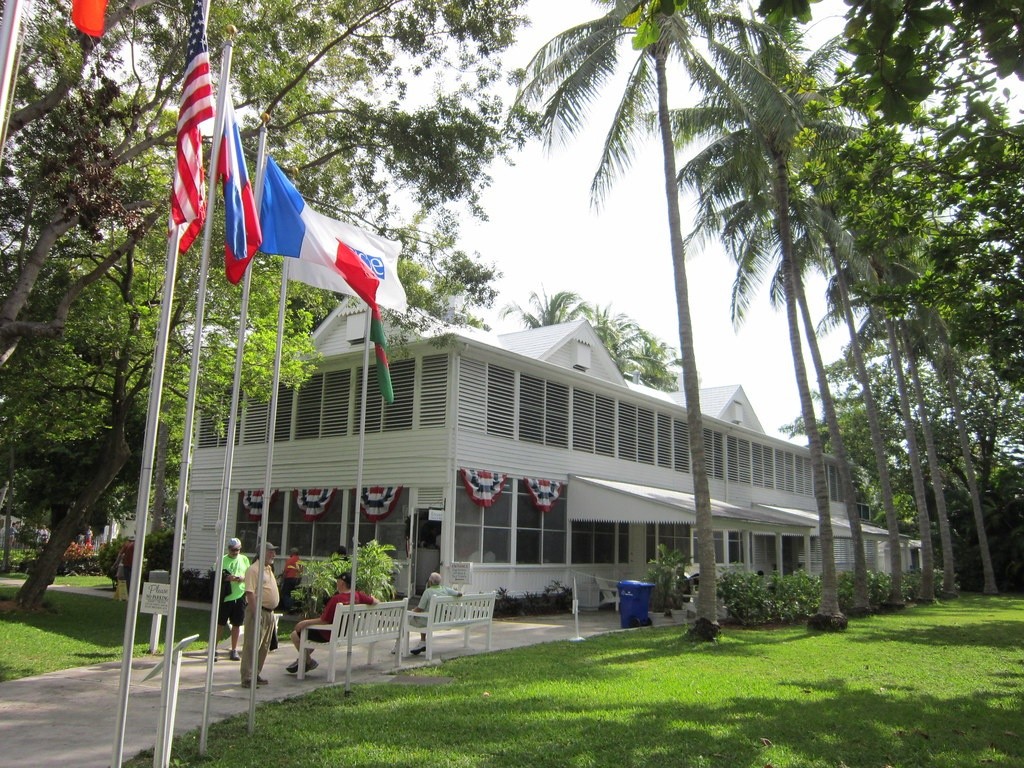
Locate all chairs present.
[595,577,621,611]
[677,573,701,602]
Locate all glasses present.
[230,548,242,553]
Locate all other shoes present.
[410,641,426,655]
[305,659,319,672]
[257,675,268,685]
[241,680,260,690]
[286,658,299,672]
[230,650,240,661]
[391,643,396,653]
[215,652,218,662]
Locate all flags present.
[72,0,108,36]
[370,305,395,402]
[258,155,380,311]
[215,58,262,287]
[289,210,407,314]
[171,0,215,254]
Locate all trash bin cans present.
[616,579,655,628]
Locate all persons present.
[37,524,48,545]
[68,526,93,556]
[111,536,135,596]
[240,542,280,688]
[322,546,349,609]
[10,522,16,549]
[459,538,496,563]
[214,538,250,662]
[286,572,380,673]
[284,547,303,614]
[410,572,462,655]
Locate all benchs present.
[402,591,496,662]
[297,597,409,682]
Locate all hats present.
[255,542,279,557]
[334,571,352,586]
[128,536,136,540]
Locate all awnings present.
[566,475,816,535]
[752,503,910,543]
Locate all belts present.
[261,606,276,613]
